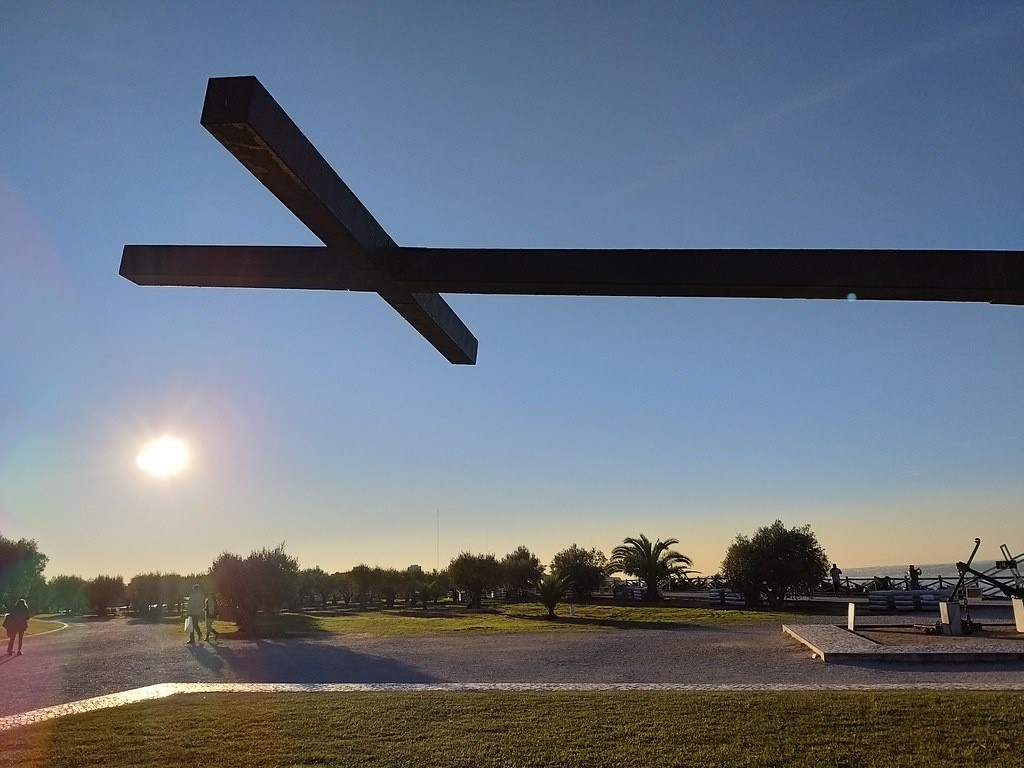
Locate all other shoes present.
[187,639,195,644]
[199,633,202,640]
[17,651,23,656]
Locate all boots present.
[211,629,219,641]
[204,634,210,641]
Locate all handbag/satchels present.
[184,616,193,634]
[3,614,10,630]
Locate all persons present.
[830,564,842,597]
[186,583,204,644]
[6,599,30,656]
[203,592,220,642]
[908,564,922,590]
